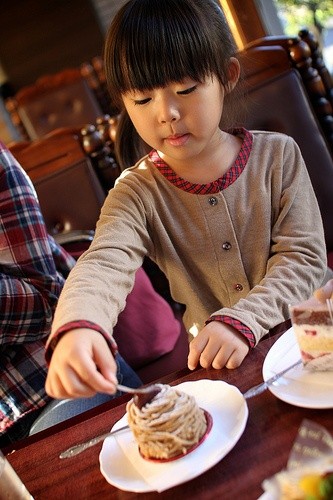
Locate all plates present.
[262,326,333,409]
[99,379,249,493]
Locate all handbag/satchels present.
[113,268,181,373]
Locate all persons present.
[43,0,328,398]
[0,141,140,446]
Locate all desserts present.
[288,278,333,374]
[272,417,333,500]
[125,383,208,461]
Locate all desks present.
[2,320,333,500]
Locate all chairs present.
[6,126,107,233]
[114,28,333,255]
[15,67,104,139]
[60,236,192,381]
